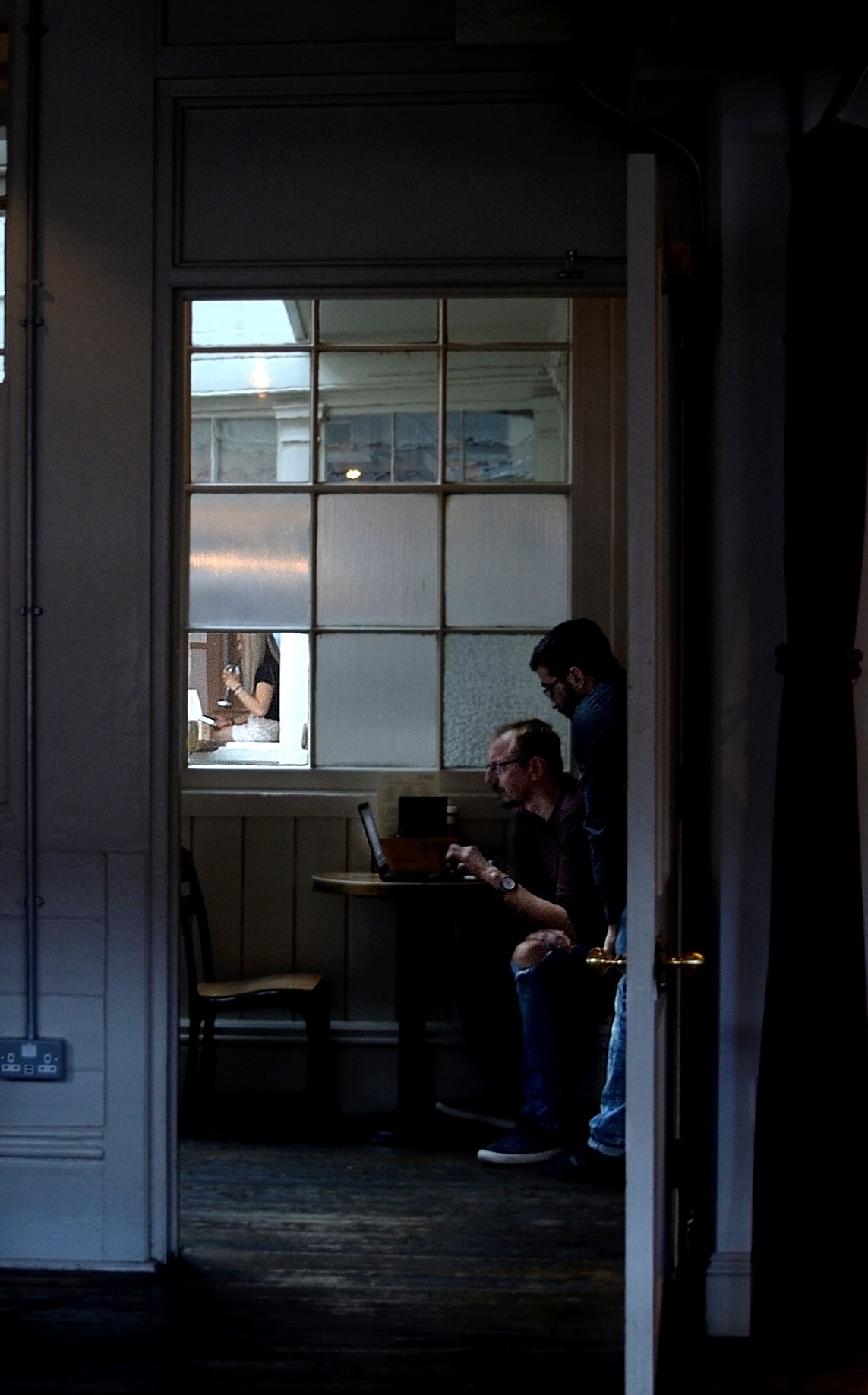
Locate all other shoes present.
[541,1147,625,1189]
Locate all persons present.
[432,718,608,1166]
[529,614,630,1177]
[221,631,280,743]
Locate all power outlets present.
[0,1037,66,1080]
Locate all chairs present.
[180,847,328,1150]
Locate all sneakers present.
[477,1125,568,1163]
[435,1091,522,1129]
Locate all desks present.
[310,871,488,1147]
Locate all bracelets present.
[233,684,244,696]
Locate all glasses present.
[485,757,522,776]
[543,677,562,700]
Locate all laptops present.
[357,802,439,883]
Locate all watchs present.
[496,873,519,898]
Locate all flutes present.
[214,656,242,707]
